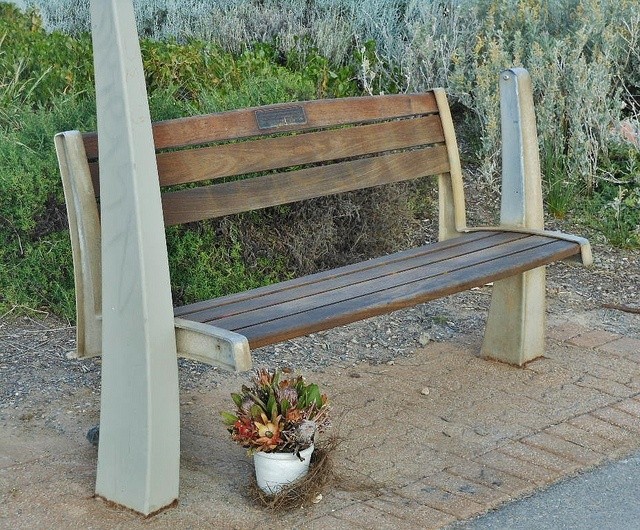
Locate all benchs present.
[53,69,594,516]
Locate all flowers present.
[221,367,334,461]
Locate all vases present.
[252,442,315,497]
